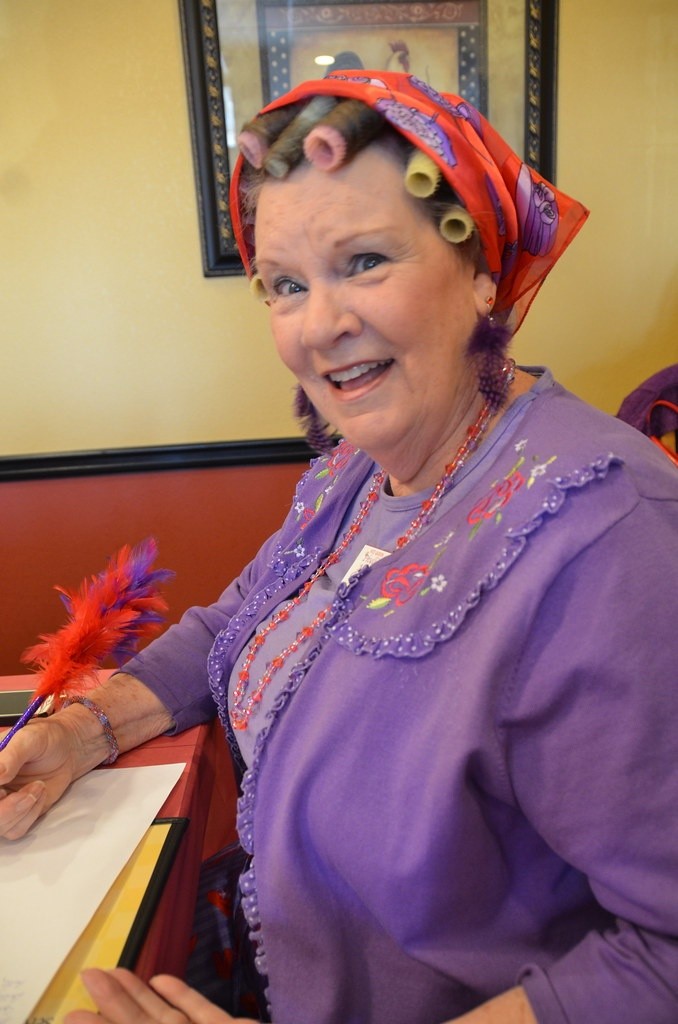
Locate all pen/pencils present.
[0,536,177,753]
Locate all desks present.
[0,669,213,1024]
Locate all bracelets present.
[60,696,120,765]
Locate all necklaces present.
[225,360,517,732]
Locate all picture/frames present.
[178,0,563,277]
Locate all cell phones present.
[0,690,56,729]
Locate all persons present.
[0,68,678,1024]
